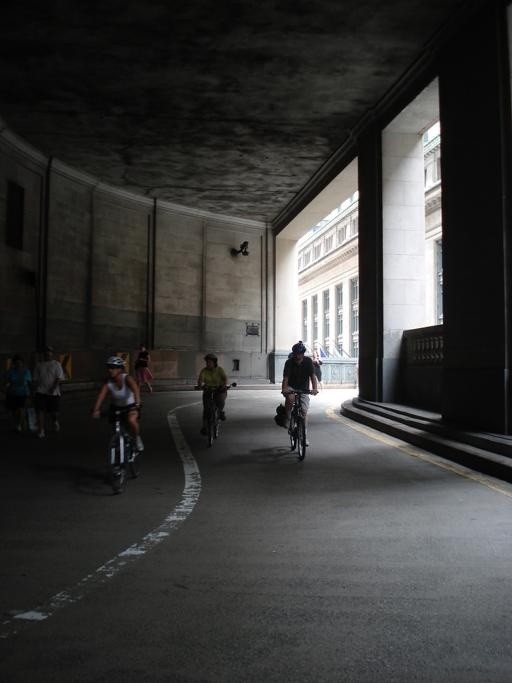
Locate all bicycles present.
[194,379,238,447]
[283,390,319,462]
[96,405,142,494]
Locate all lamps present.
[229,241,249,256]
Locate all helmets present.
[204,354,217,360]
[288,352,296,358]
[106,356,128,366]
[292,343,306,354]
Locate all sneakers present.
[38,429,46,438]
[217,411,225,421]
[200,427,208,435]
[135,435,144,451]
[113,466,121,478]
[301,439,309,446]
[283,418,290,428]
[15,423,22,432]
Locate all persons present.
[35,346,66,440]
[134,343,154,393]
[197,352,229,435]
[90,354,145,480]
[281,339,318,447]
[5,353,34,433]
[29,350,43,370]
